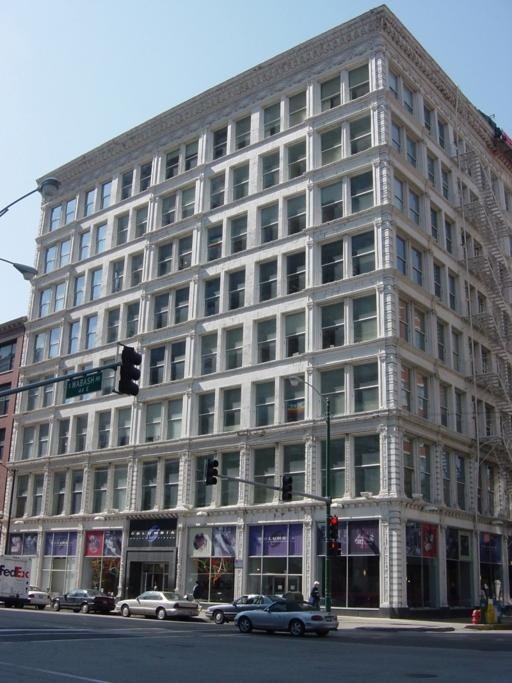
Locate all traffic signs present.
[66,373,105,397]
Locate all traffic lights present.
[283,475,293,501]
[329,516,338,538]
[336,541,341,556]
[327,541,339,557]
[120,347,142,397]
[206,459,219,486]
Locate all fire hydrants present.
[470,609,481,624]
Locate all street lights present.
[1,175,62,217]
[0,256,39,282]
[288,374,331,611]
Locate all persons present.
[309,580,321,611]
[193,580,202,610]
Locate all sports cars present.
[235,599,338,636]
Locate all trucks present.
[0,554,32,608]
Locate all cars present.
[205,593,285,623]
[28,585,51,610]
[116,591,200,620]
[51,588,116,614]
